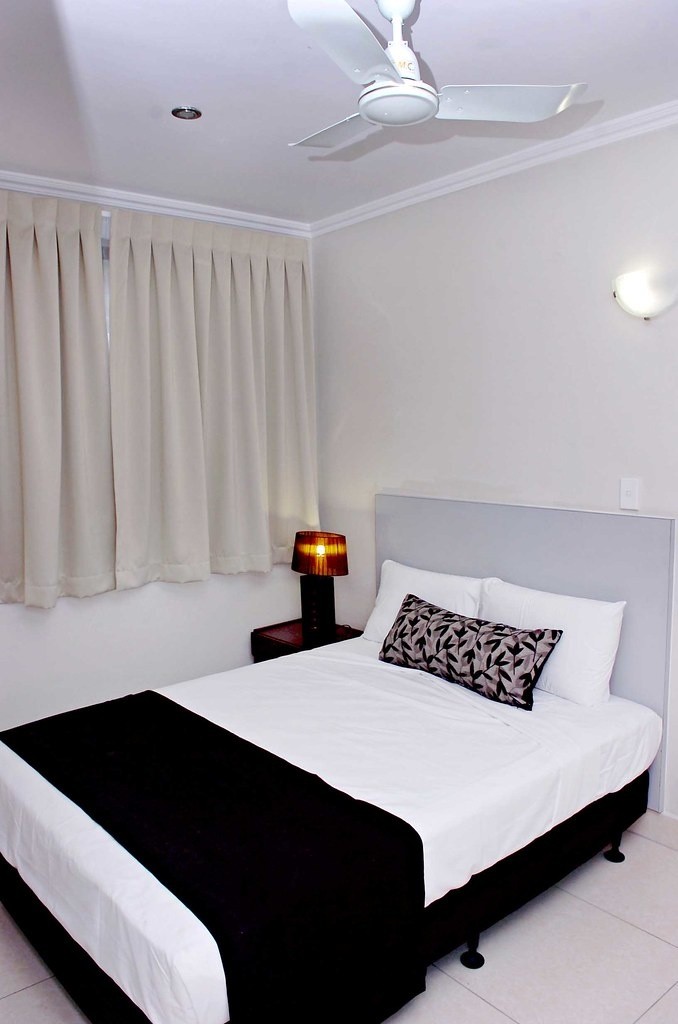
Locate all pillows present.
[360,558,482,642]
[479,576,626,710]
[378,594,564,711]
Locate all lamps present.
[291,531,349,642]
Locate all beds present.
[0,491,674,1024]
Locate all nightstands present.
[250,617,363,663]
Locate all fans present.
[287,0,588,148]
